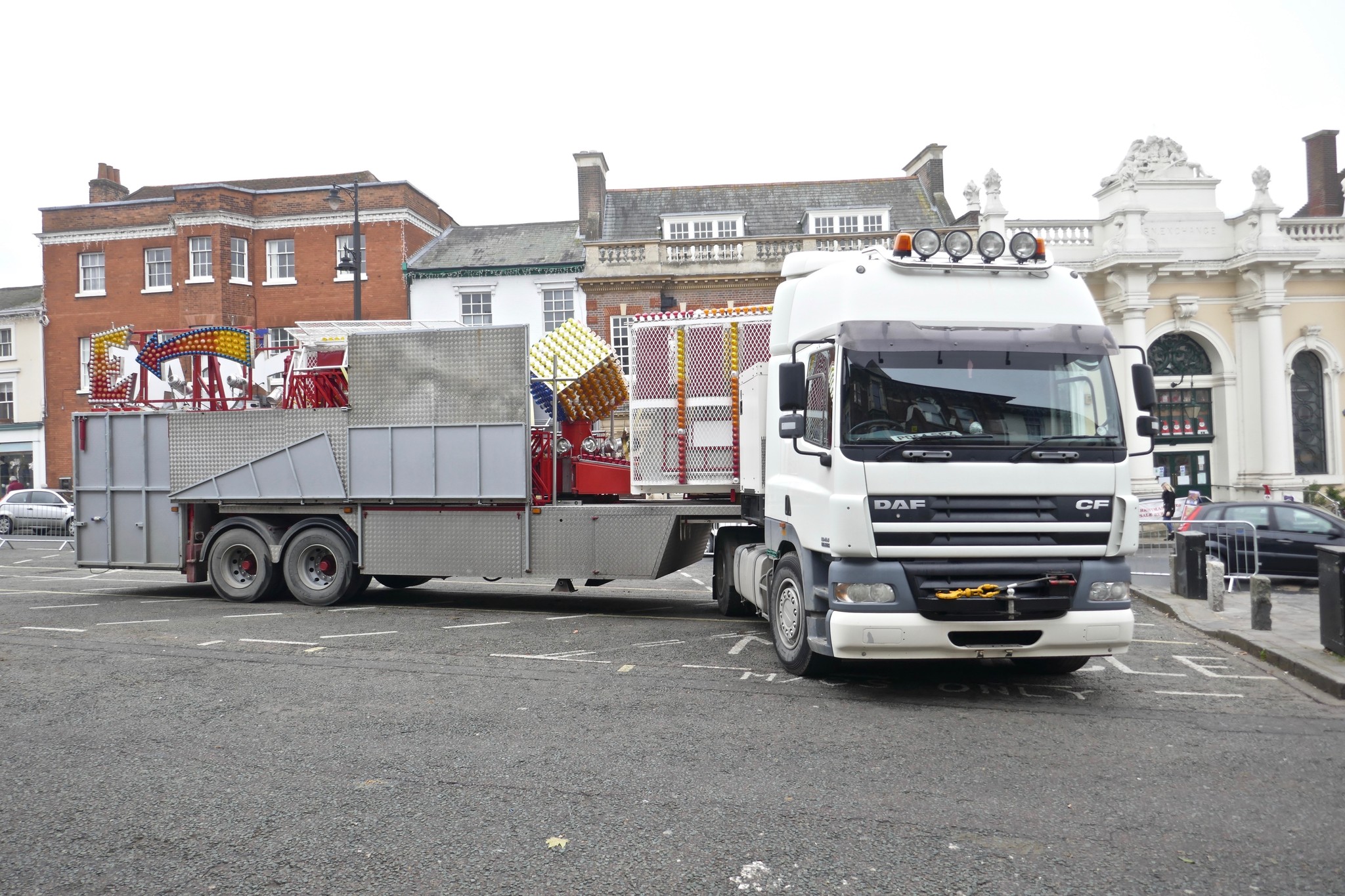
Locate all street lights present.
[321,179,362,320]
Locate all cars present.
[0,488,75,536]
[1174,499,1345,587]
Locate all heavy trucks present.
[69,229,1162,681]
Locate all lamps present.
[1171,366,1201,420]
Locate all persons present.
[1161,482,1175,541]
[8,476,24,493]
[0,456,9,497]
[22,465,30,489]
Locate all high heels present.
[1164,535,1173,541]
[1170,531,1175,539]
